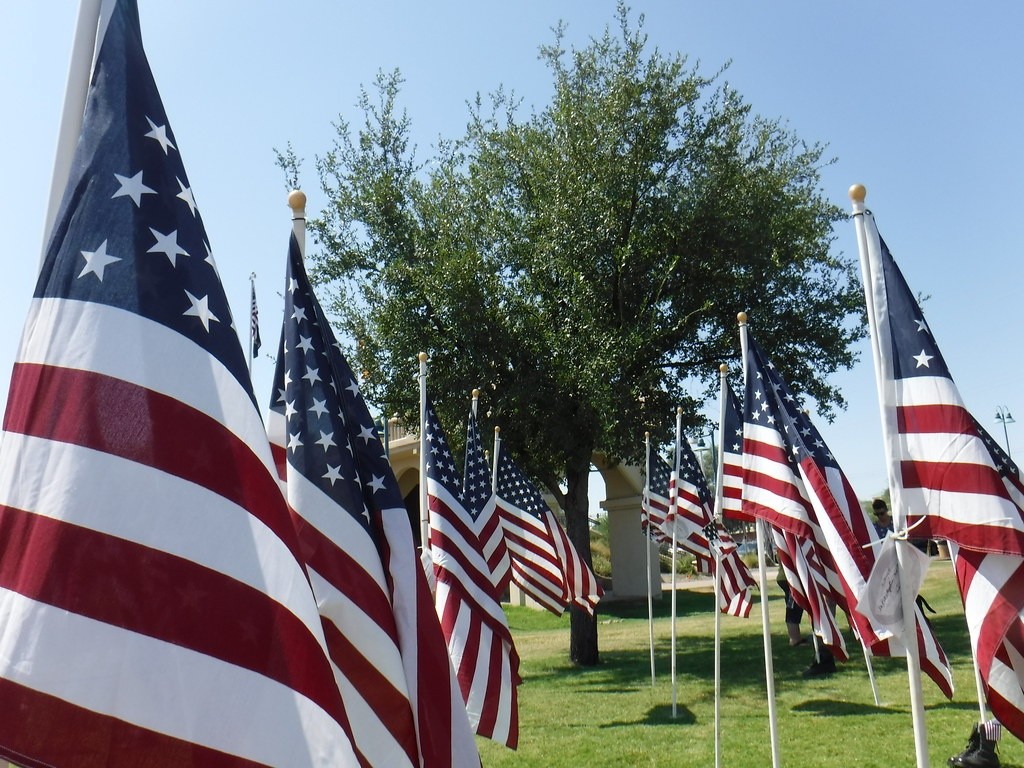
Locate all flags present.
[640,326,956,704]
[251,280,263,359]
[419,397,524,750]
[0,1,368,768]
[267,231,482,768]
[857,206,1024,739]
[493,439,605,618]
[463,408,514,599]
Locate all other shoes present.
[792,638,808,647]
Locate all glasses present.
[873,511,887,516]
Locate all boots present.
[953,722,1000,768]
[946,722,980,768]
[803,646,836,676]
